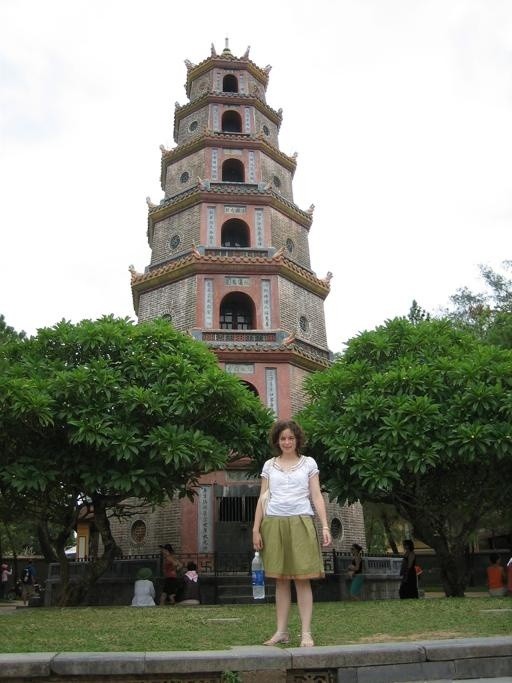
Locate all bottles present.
[252,552,265,599]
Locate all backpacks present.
[21,567,33,584]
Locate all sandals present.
[263,631,290,646]
[300,631,314,647]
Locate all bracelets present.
[323,527,331,531]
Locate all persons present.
[347,544,362,600]
[486,552,512,597]
[131,568,156,606]
[252,421,332,647]
[1,564,13,600]
[399,540,418,599]
[158,543,184,605]
[22,559,37,605]
[175,562,201,605]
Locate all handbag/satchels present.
[415,566,423,575]
[261,488,271,519]
[345,571,354,579]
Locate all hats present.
[136,568,152,580]
[159,543,173,551]
